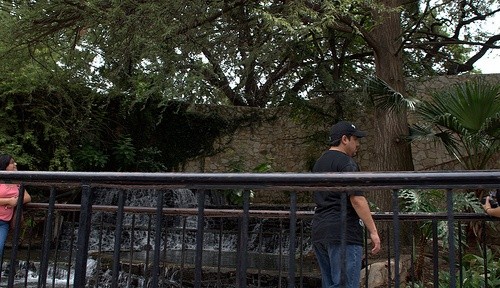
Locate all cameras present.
[483,192,497,208]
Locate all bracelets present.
[486,208,491,213]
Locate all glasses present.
[9,160,16,164]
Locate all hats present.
[329,121,368,141]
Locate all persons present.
[482,195,500,218]
[308,120,380,288]
[0,153,32,283]
